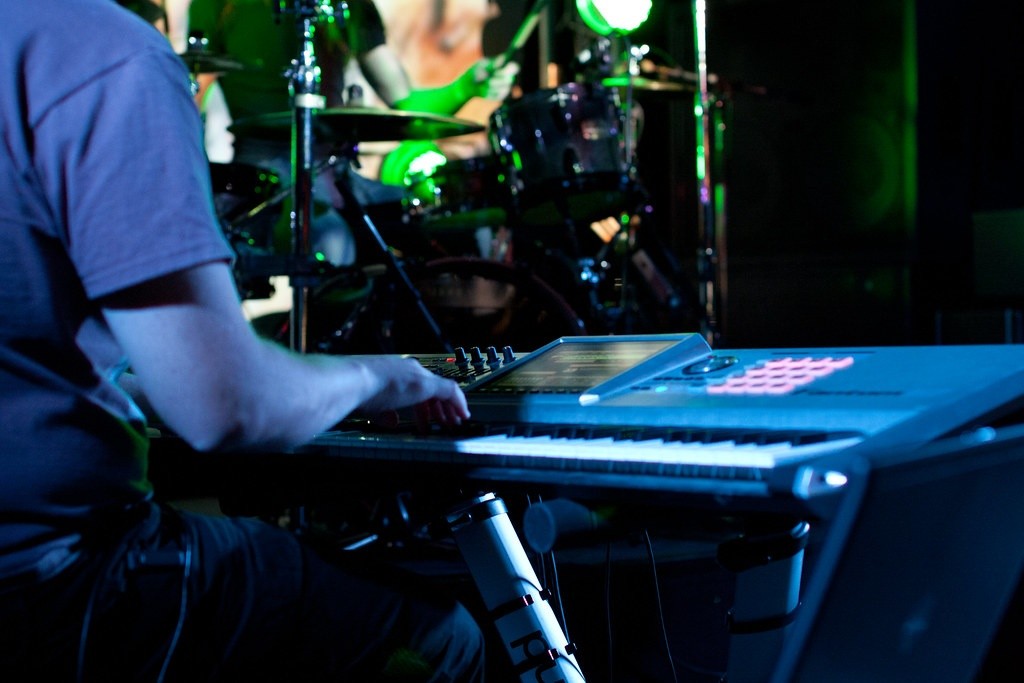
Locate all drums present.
[287,256,593,356]
[202,155,286,302]
[397,204,524,332]
[485,74,640,204]
[402,153,513,218]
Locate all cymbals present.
[184,49,276,78]
[223,104,490,144]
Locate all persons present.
[0,0,482,683]
[187,0,521,354]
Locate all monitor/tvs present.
[493,339,684,387]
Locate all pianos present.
[140,326,1024,510]
